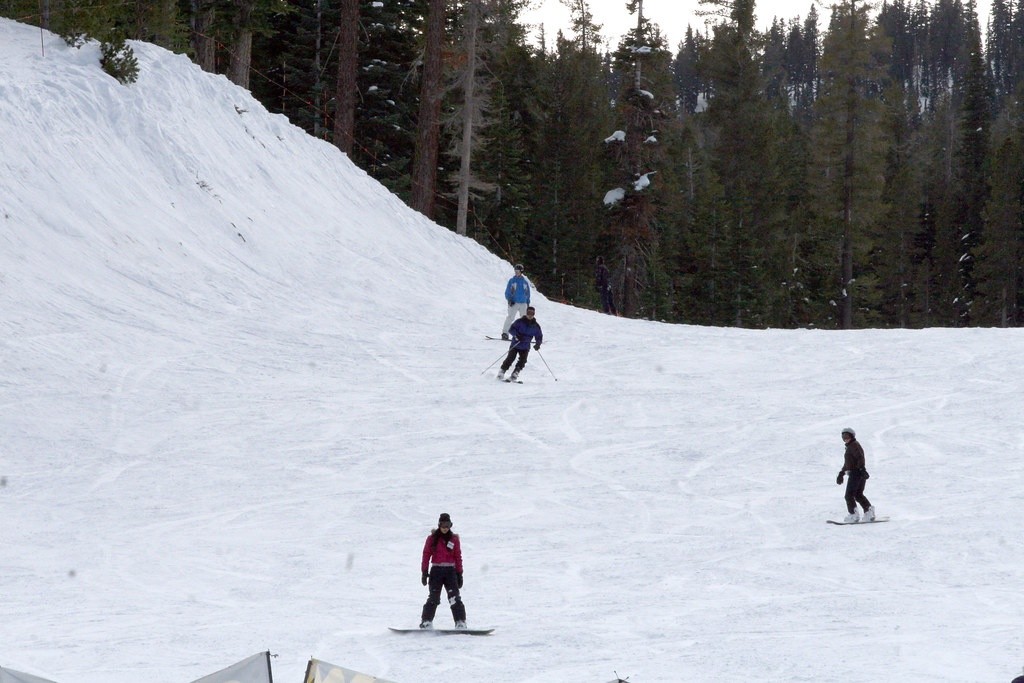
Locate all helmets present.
[514,264,524,272]
[842,428,856,437]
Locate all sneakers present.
[497,368,505,380]
[420,620,433,629]
[844,507,860,523]
[454,620,467,629]
[510,371,519,380]
[501,333,508,340]
[862,506,876,522]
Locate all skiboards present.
[492,373,529,387]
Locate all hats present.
[438,513,453,528]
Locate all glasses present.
[527,311,535,316]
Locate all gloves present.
[513,334,521,342]
[508,298,515,307]
[836,471,846,485]
[533,344,540,351]
[457,572,463,588]
[422,570,431,586]
[859,467,870,479]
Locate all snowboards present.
[823,514,892,528]
[485,334,550,346]
[385,623,501,640]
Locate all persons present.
[837,428,876,522]
[498,307,543,381]
[420,513,467,629]
[501,264,530,340]
